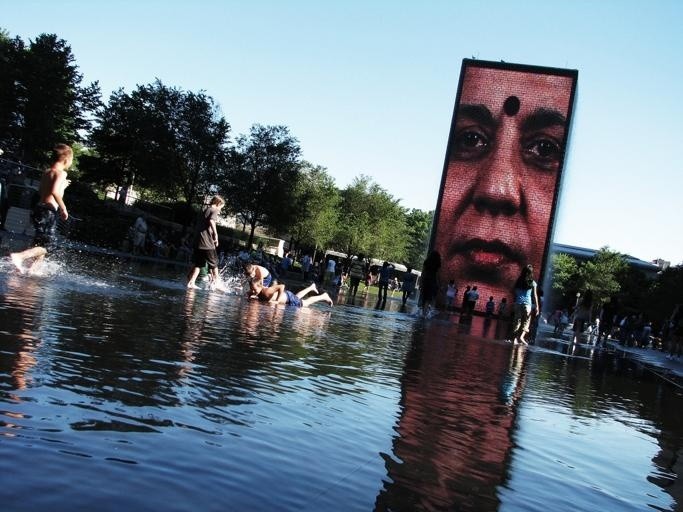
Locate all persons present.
[445,280,458,311]
[510,264,539,346]
[243,264,333,308]
[485,296,495,318]
[6,281,48,388]
[460,286,479,315]
[301,253,373,292]
[10,143,74,279]
[495,340,528,407]
[184,194,231,295]
[132,211,192,257]
[219,238,293,278]
[372,317,529,512]
[496,298,508,314]
[378,262,395,299]
[554,293,682,363]
[430,64,572,317]
[400,268,415,305]
[391,278,399,297]
[419,251,442,316]
